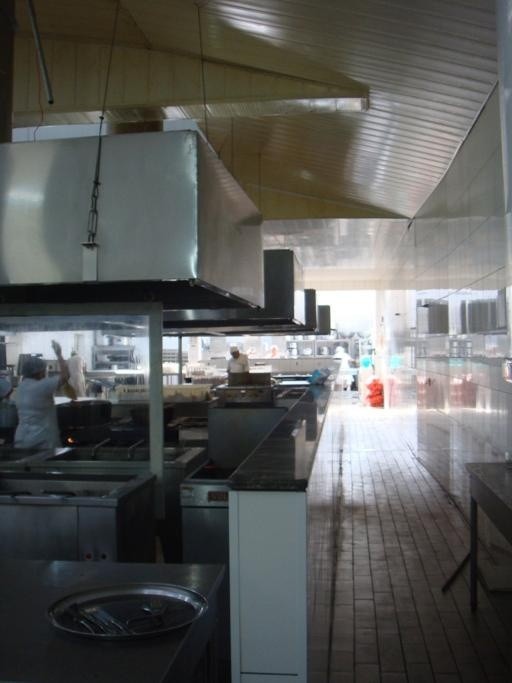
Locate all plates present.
[46,582,209,642]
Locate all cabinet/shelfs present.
[284,338,354,359]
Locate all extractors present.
[0,129,331,337]
[0,127,333,336]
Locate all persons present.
[14,339,70,449]
[66,350,88,398]
[226,346,250,373]
[265,345,280,358]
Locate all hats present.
[230,346,238,353]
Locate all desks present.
[464,460,512,626]
